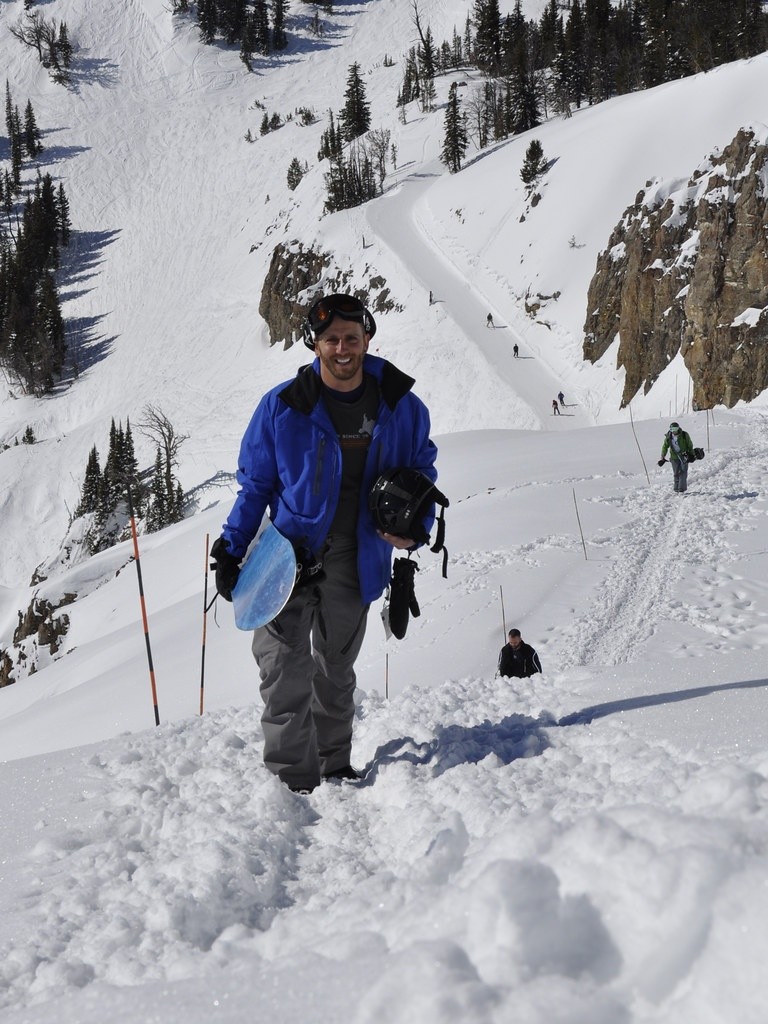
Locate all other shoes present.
[288,765,363,795]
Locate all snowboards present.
[676,448,705,464]
[231,513,297,631]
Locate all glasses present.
[308,294,365,332]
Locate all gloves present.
[389,557,420,640]
[209,537,242,602]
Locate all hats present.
[670,422,680,432]
[303,305,376,352]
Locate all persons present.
[558,391,564,405]
[498,629,543,678]
[553,400,560,415]
[487,313,494,327]
[660,422,694,492]
[513,344,518,358]
[206,294,438,794]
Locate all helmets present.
[367,468,450,544]
